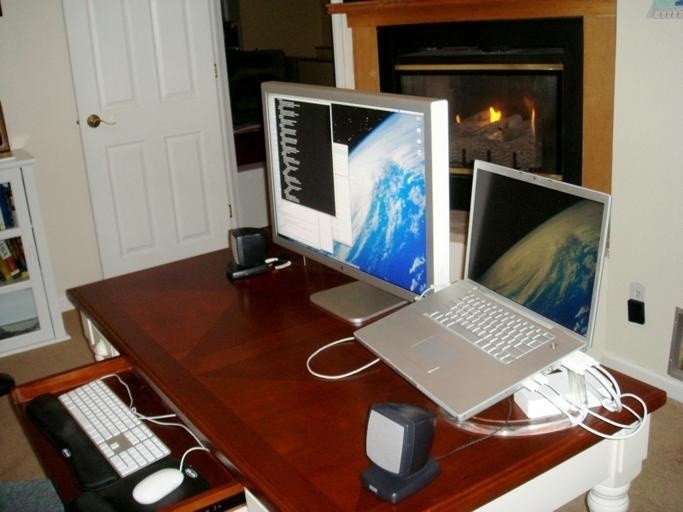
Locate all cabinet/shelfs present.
[0,148,71,358]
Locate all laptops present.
[354,157,610,424]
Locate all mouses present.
[130,467,185,506]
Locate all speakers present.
[226,227,268,279]
[360,401,441,502]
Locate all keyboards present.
[57,379,173,480]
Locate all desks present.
[8,226,667,512]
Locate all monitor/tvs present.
[261,80,449,323]
